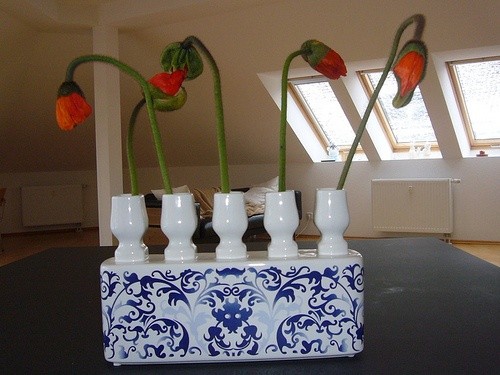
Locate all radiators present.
[19,184,88,233]
[371,178,461,245]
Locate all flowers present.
[127,69,188,195]
[160,36,229,194]
[56,55,173,194]
[336,14,427,190]
[279,40,347,192]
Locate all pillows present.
[249,176,279,192]
[245,187,273,216]
[151,185,196,203]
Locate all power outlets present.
[307,212,313,221]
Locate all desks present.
[0,236,500,374]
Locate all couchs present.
[144,188,302,246]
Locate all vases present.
[99,188,365,366]
